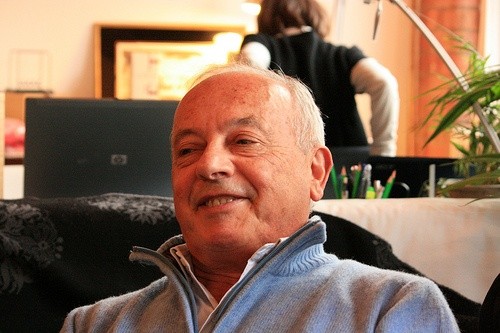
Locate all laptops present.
[23,98,180,198]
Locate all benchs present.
[0,155,500,333]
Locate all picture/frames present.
[93,22,247,100]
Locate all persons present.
[59,64,462,333]
[240,0,399,157]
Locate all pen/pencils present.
[331,165,396,199]
